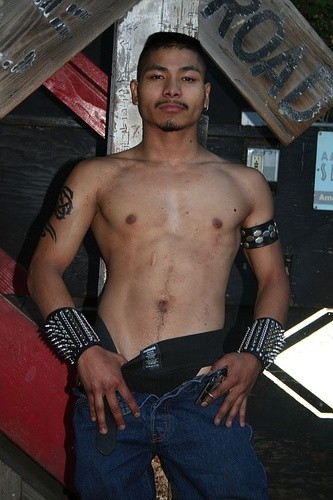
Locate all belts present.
[94,403,116,456]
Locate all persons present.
[26,31,290,500]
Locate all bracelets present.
[45,307,102,366]
[236,317,286,376]
